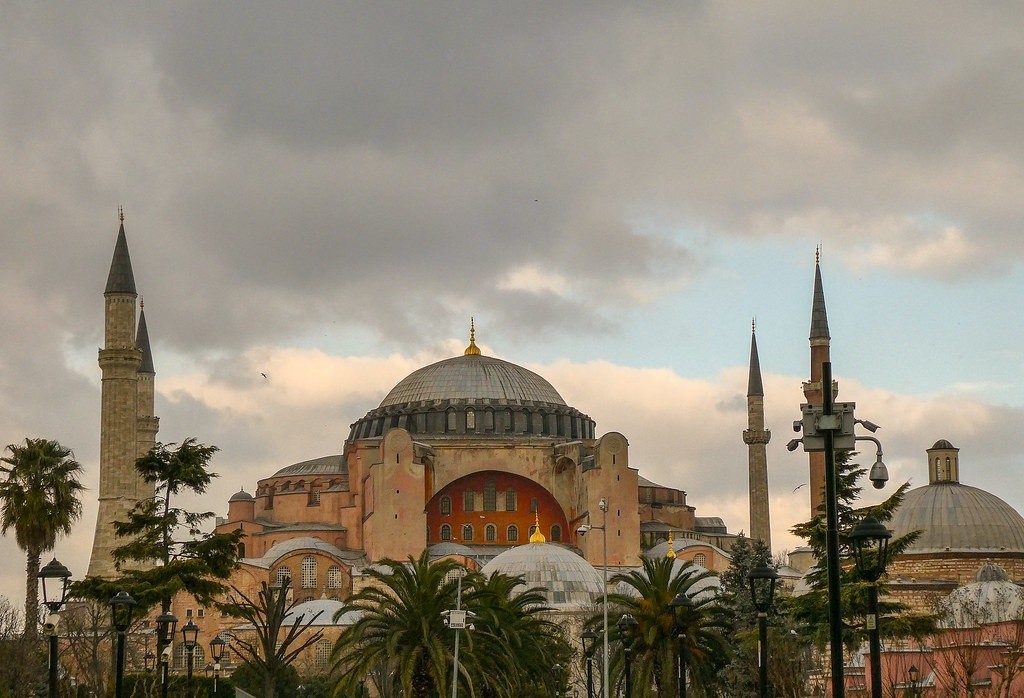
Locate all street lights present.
[578,497,610,698]
[746,558,780,698]
[669,593,696,698]
[825,436,892,698]
[209,635,226,698]
[155,610,178,698]
[580,625,599,698]
[844,506,891,698]
[180,620,200,698]
[36,552,72,698]
[616,614,640,698]
[143,652,156,674]
[108,592,136,698]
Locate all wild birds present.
[792,483,807,493]
[261,372,267,379]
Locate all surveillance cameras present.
[467,611,476,617]
[440,610,450,616]
[43,614,61,631]
[868,461,889,489]
[444,619,448,625]
[578,527,588,535]
[214,664,221,672]
[469,625,475,632]
[161,647,172,658]
